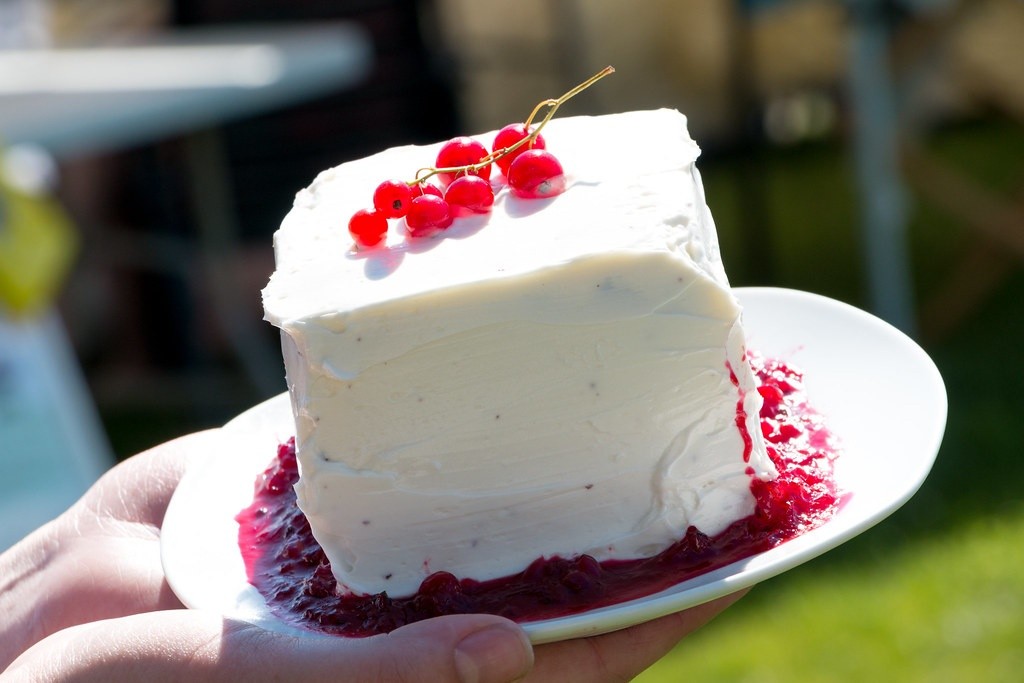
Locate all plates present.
[158,288,947,644]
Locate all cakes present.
[260,67,777,627]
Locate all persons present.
[0,427,756,683]
